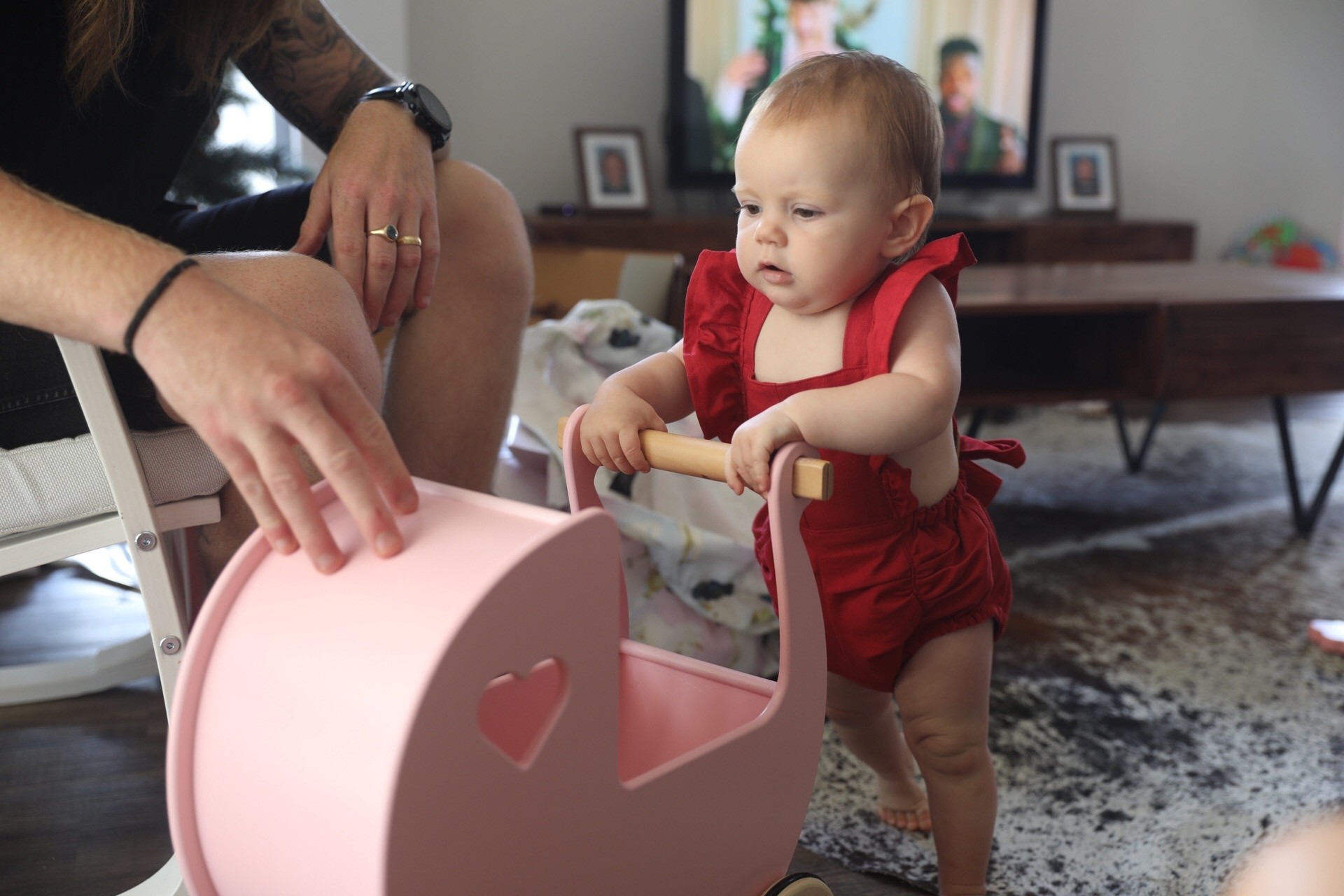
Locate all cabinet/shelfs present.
[525,196,1200,402]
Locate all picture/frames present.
[571,124,654,215]
[1050,135,1122,218]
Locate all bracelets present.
[123,256,202,362]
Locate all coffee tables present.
[941,259,1344,551]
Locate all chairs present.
[0,324,228,740]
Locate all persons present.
[578,46,1031,896]
[710,0,863,138]
[598,148,631,195]
[0,0,543,579]
[1071,154,1102,197]
[927,31,1027,180]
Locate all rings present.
[366,223,399,244]
[395,235,423,248]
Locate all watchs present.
[353,80,453,154]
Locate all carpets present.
[771,403,1344,896]
